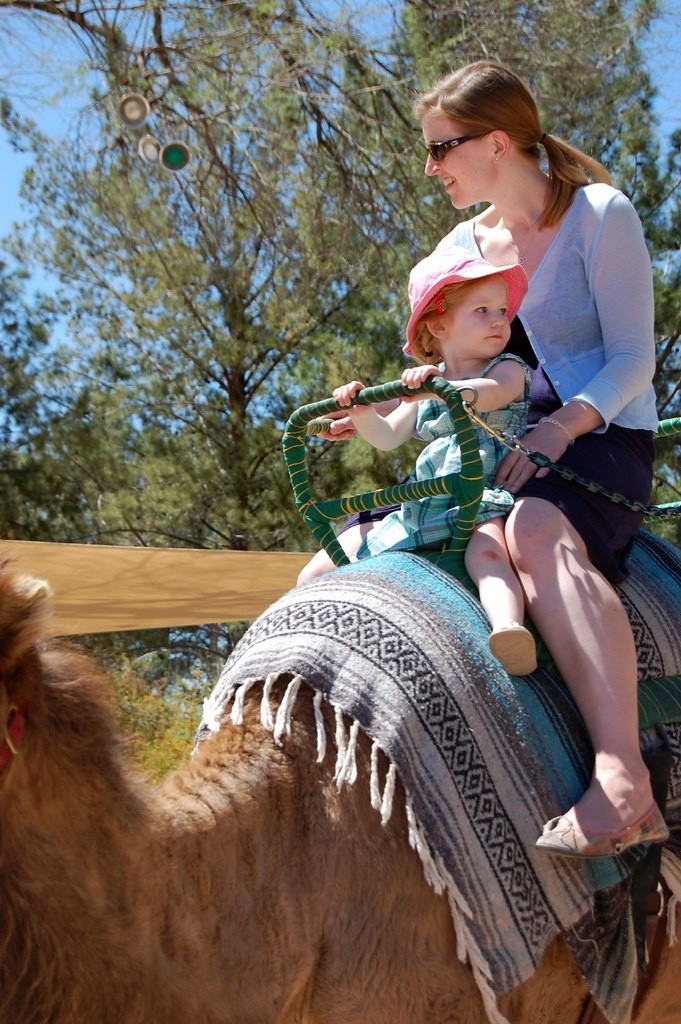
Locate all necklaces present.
[505,235,533,265]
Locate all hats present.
[403,247,529,358]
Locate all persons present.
[330,246,535,676]
[297,59,668,858]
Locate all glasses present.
[427,133,488,162]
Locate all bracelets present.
[538,417,575,444]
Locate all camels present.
[0,527,681,1023]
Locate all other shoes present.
[489,621,537,676]
[535,801,670,858]
